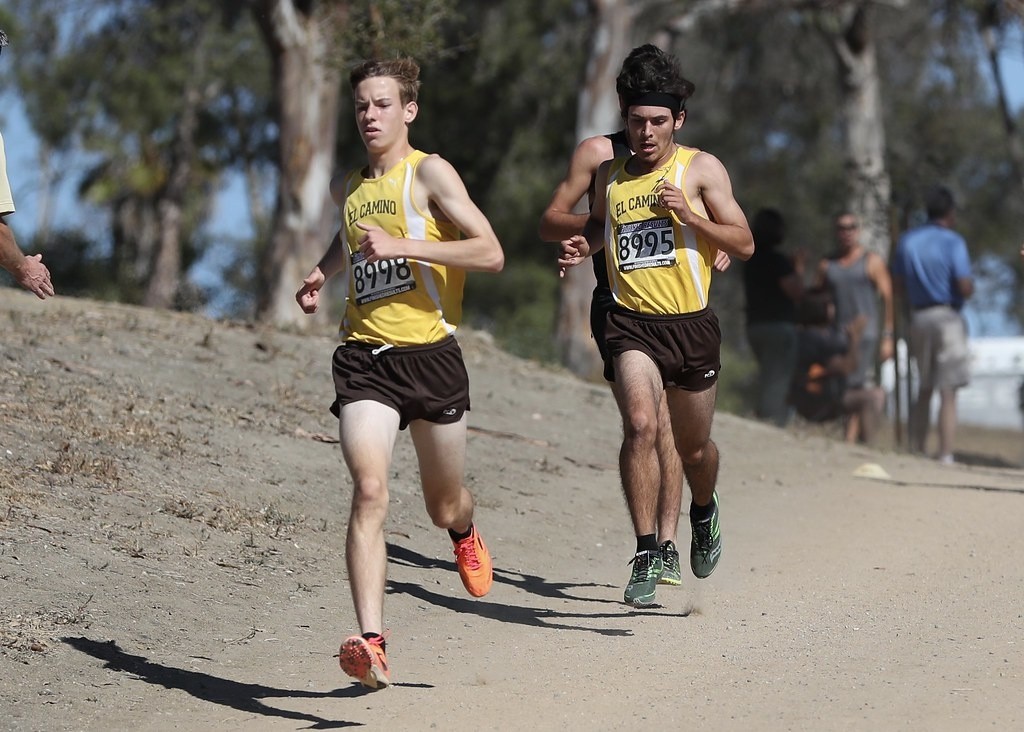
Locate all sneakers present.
[339,636,390,690]
[690,491,723,580]
[624,551,665,606]
[658,539,681,586]
[452,522,493,597]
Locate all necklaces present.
[653,146,678,183]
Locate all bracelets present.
[881,331,893,337]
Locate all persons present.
[546,41,735,593]
[743,205,812,431]
[889,188,972,464]
[794,243,842,435]
[0,130,54,300]
[293,57,504,690]
[551,56,759,617]
[819,212,893,447]
